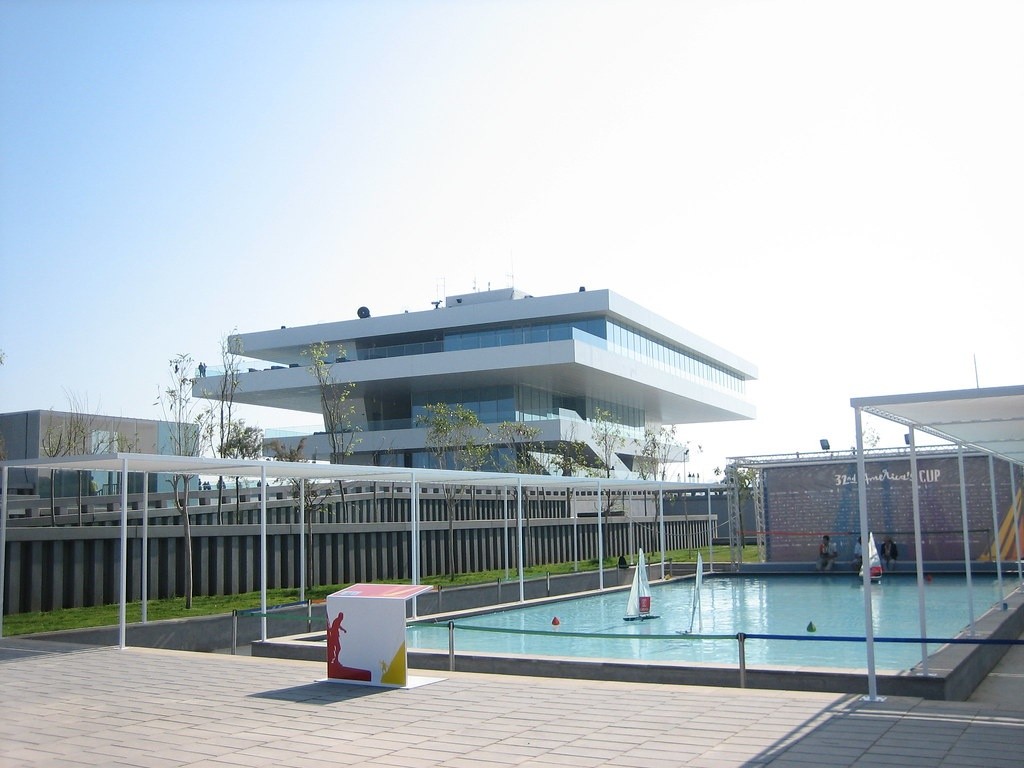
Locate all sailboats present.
[622,547,660,621]
[674,552,705,636]
[858,531,884,582]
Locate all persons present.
[853,537,862,571]
[881,536,898,571]
[815,535,838,571]
